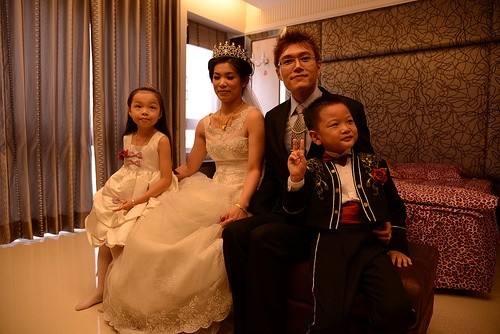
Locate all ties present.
[289,104,306,155]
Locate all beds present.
[383,159,498,296]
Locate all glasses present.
[278,55,316,69]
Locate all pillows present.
[389,162,460,180]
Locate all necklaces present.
[289,113,307,134]
[220,100,244,132]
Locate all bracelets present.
[132,199,136,207]
[235,204,248,214]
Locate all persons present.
[75,87,179,311]
[284,94,412,334]
[221,31,392,334]
[99,42,267,334]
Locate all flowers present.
[117,149,130,161]
[370,168,389,186]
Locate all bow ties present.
[322,152,353,167]
